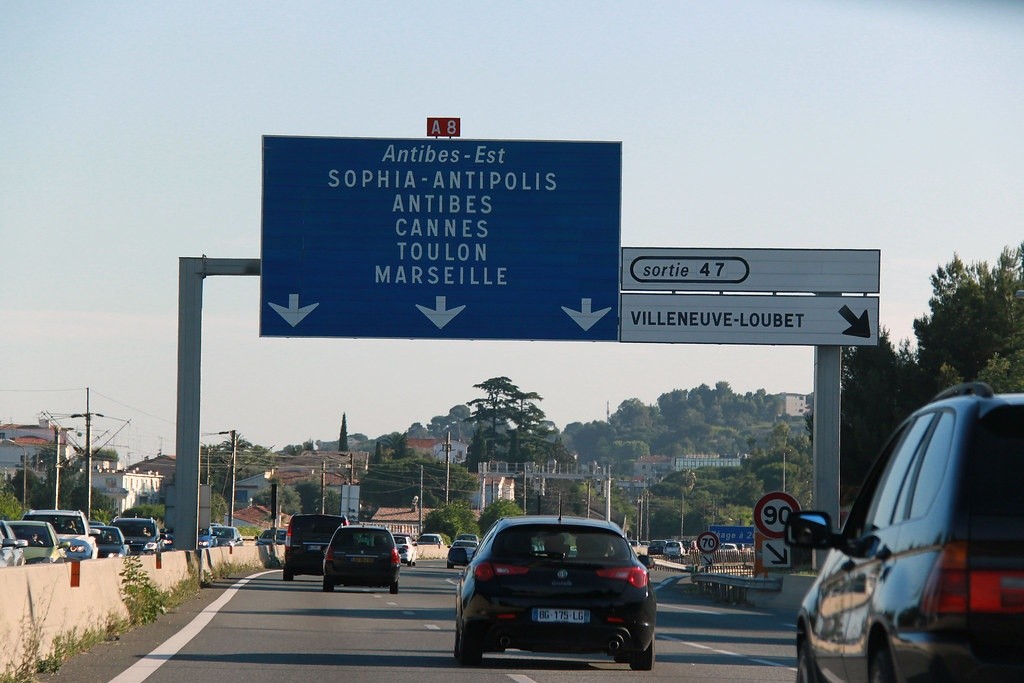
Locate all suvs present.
[782,381,1024,683]
[282,514,351,582]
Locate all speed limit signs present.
[752,490,802,540]
[696,531,720,554]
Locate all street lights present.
[218,429,236,527]
[71,412,105,521]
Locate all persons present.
[143,527,151,536]
[544,535,565,560]
[797,527,812,542]
[65,520,77,533]
[107,535,112,541]
[29,531,43,545]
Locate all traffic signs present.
[618,291,880,350]
[259,134,623,341]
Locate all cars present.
[628,538,755,560]
[446,534,480,568]
[254,528,288,546]
[0,507,244,567]
[320,522,402,595]
[416,533,444,545]
[393,533,417,566]
[452,513,658,673]
